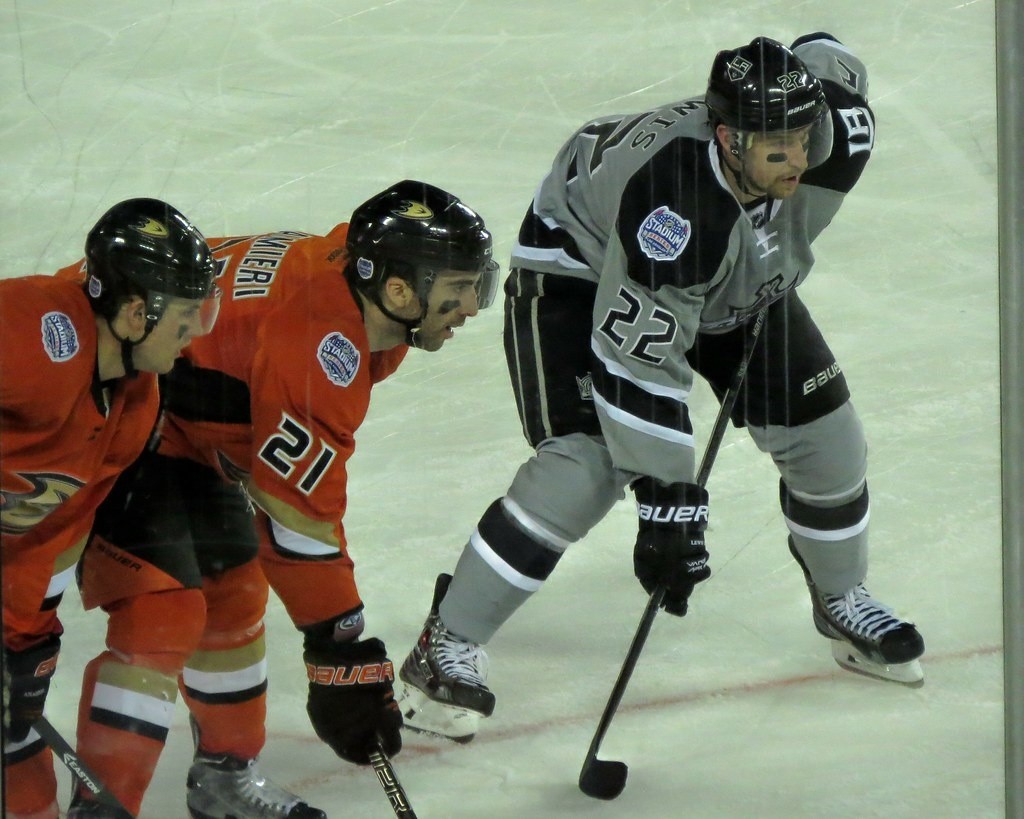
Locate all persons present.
[52,179,502,819]
[0,197,225,819]
[391,32,926,746]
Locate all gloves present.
[303,637,404,765]
[629,476,712,617]
[1,635,60,743]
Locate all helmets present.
[705,36,826,151]
[84,199,216,316]
[346,180,494,295]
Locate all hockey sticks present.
[370,745,416,819]
[33,714,133,819]
[577,303,777,801]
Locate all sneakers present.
[186,713,326,819]
[395,572,495,744]
[788,533,924,682]
